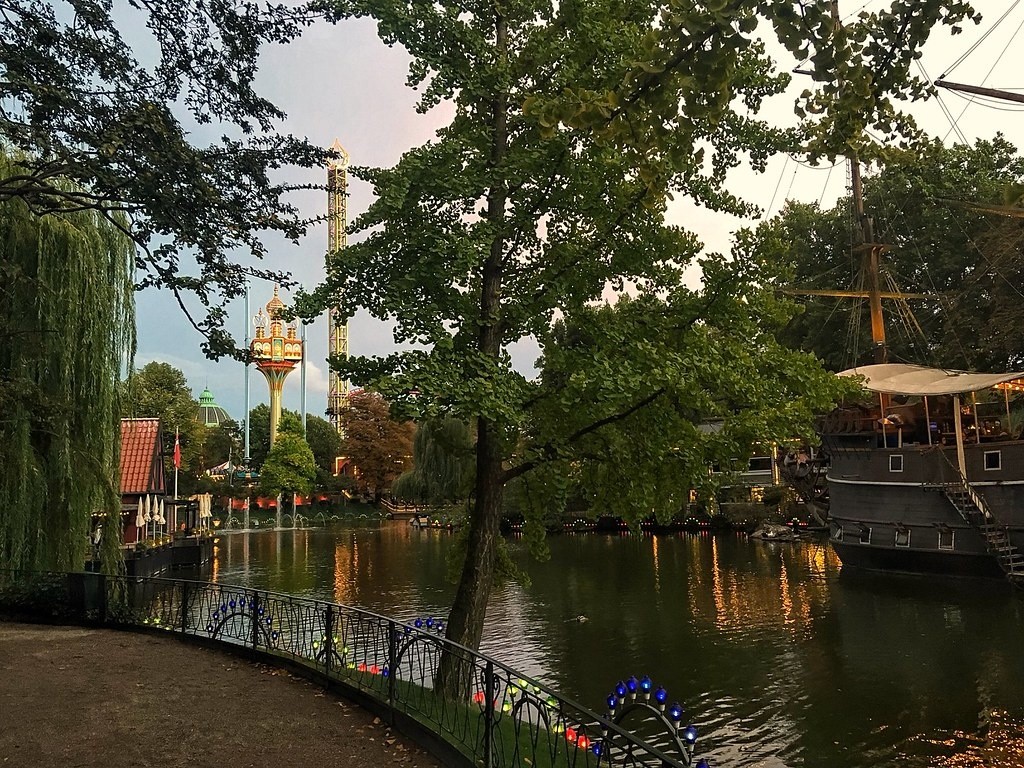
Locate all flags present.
[173,434,181,470]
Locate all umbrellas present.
[136,494,166,543]
[199,492,213,536]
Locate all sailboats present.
[764,0,1024,591]
